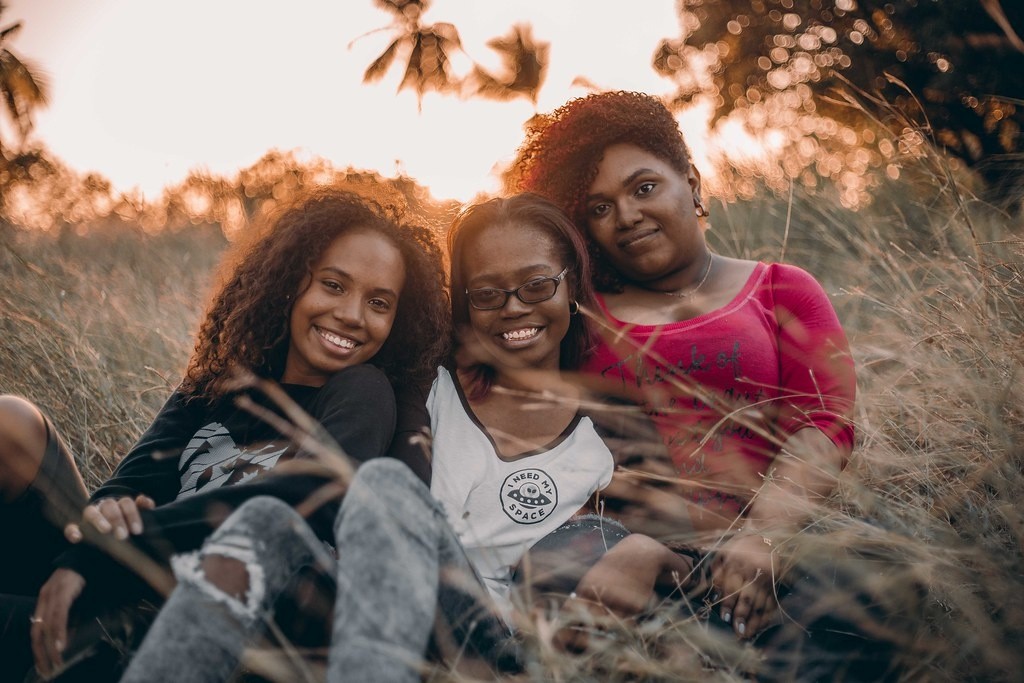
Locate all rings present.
[570,592,578,600]
[31,617,42,623]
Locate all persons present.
[115,195,704,683]
[1,188,452,683]
[515,90,895,682]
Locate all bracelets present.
[738,526,783,564]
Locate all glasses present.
[464,264,571,310]
